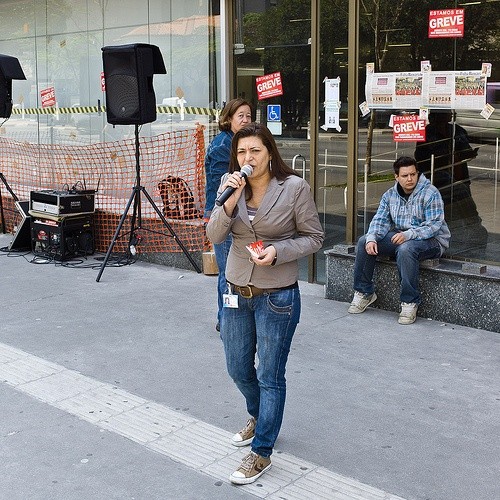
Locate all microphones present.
[216,164,253,206]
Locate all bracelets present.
[271,256,277,266]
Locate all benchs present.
[324,245,500,334]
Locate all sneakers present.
[229,451,271,485]
[231,417,257,446]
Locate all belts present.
[226,282,297,298]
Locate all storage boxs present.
[29,190,95,216]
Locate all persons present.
[205,122,324,484]
[348,157,452,325]
[203,96,251,331]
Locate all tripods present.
[95,125,201,282]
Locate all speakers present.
[0,54,13,118]
[102,44,157,126]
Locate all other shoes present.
[348,291,377,314]
[398,302,418,325]
